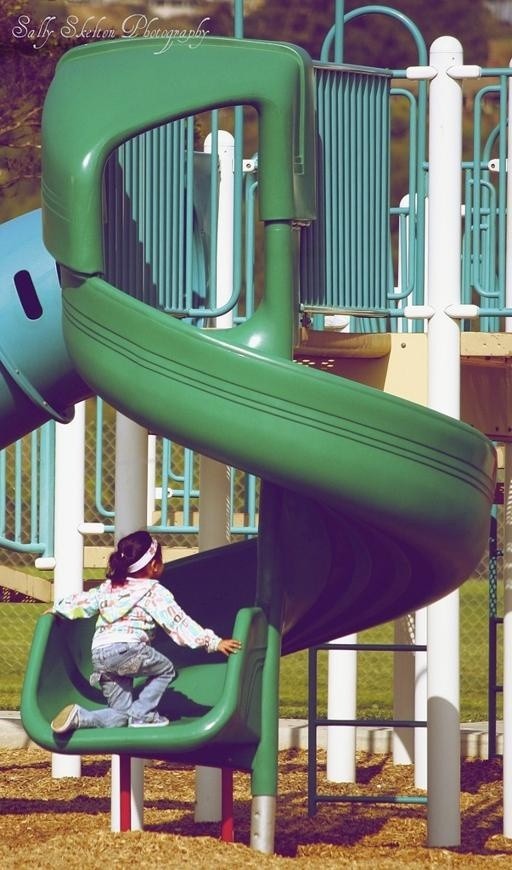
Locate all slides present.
[21,37,496,753]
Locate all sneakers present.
[50,703,80,734]
[128,711,170,728]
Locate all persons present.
[40,529,243,736]
